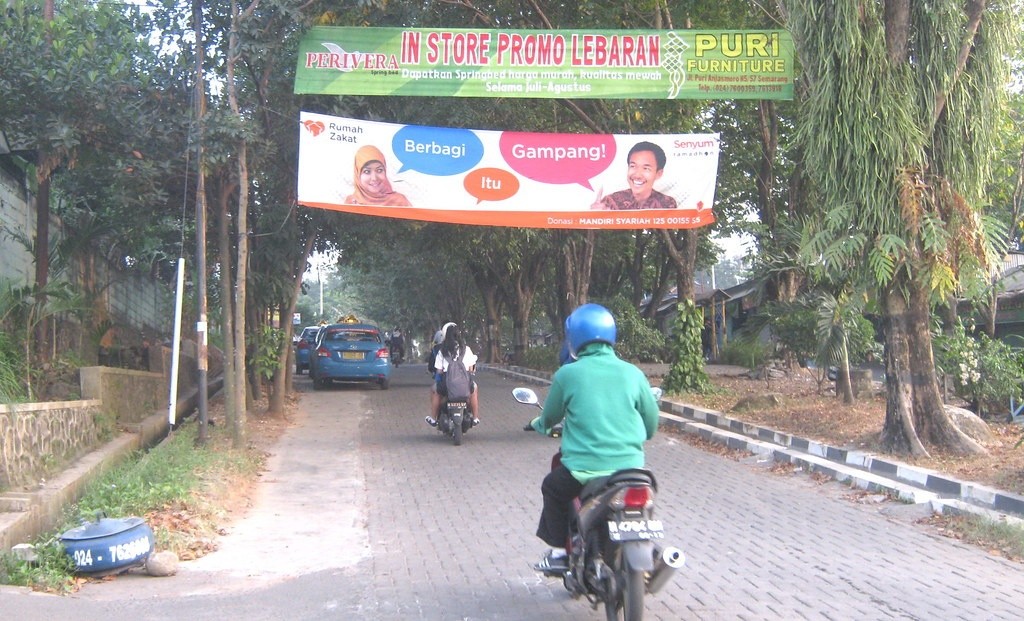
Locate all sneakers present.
[425,416,437,427]
[472,418,480,427]
[532,549,569,573]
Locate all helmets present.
[564,303,617,361]
[394,325,400,330]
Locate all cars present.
[309,315,393,390]
[293,320,328,375]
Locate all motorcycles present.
[512,387,685,621]
[385,332,405,368]
[425,355,478,446]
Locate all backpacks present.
[439,345,475,403]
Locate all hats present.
[433,330,442,345]
[441,322,458,342]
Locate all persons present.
[589,141,677,210]
[425,321,480,427]
[531,303,659,573]
[345,145,409,206]
[389,326,405,359]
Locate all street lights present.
[317,263,331,315]
